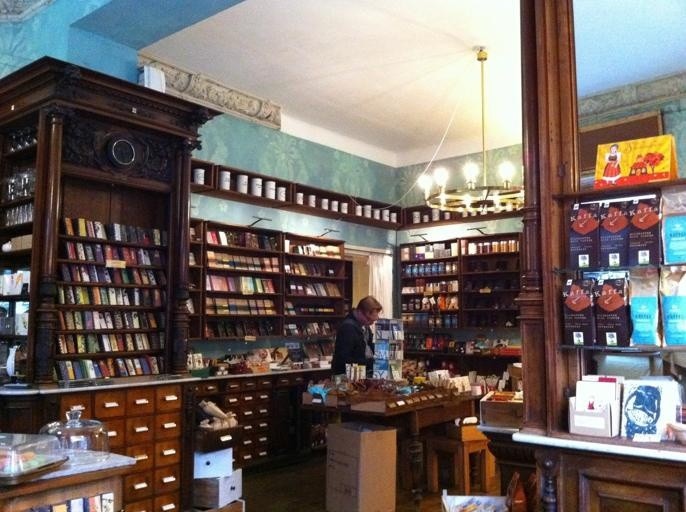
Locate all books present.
[206,223,347,355]
[54,217,167,380]
[0,269,31,337]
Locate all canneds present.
[404,262,458,277]
[477,240,519,253]
[429,314,458,329]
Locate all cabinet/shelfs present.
[1,384,185,511]
[405,204,455,231]
[188,154,296,209]
[399,230,523,420]
[515,0,686,511]
[0,446,129,512]
[185,369,333,471]
[294,181,355,223]
[282,231,348,338]
[355,195,405,230]
[456,187,529,224]
[2,49,225,393]
[183,215,207,342]
[206,220,284,339]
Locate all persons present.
[603,143,622,185]
[332,296,383,423]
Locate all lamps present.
[416,52,532,217]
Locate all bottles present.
[476,240,517,255]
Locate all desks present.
[299,376,498,512]
